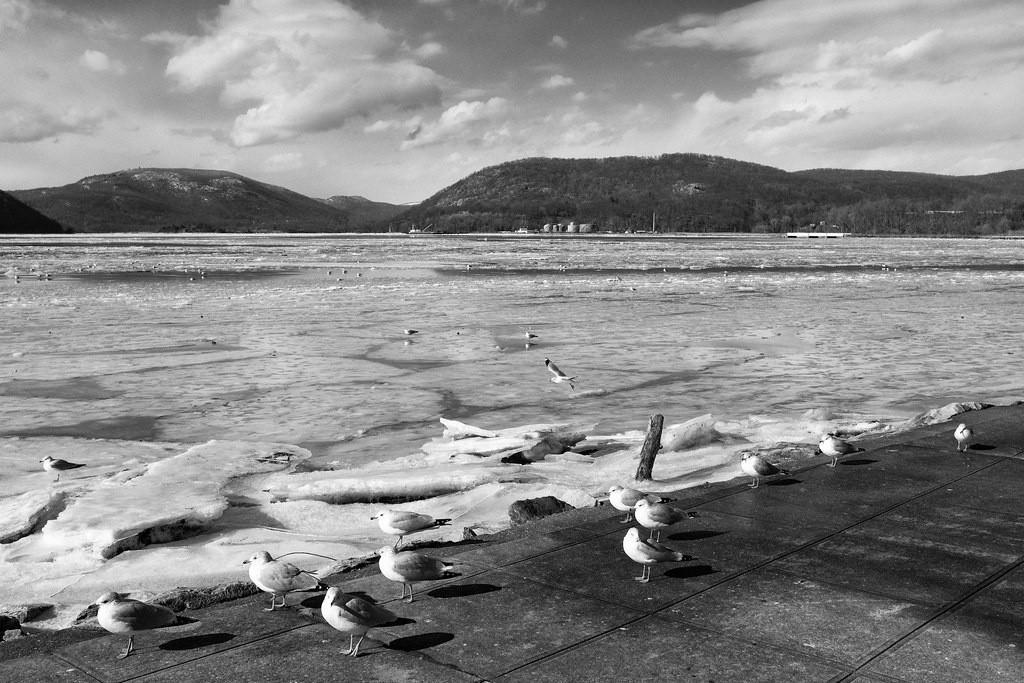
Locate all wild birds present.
[953,424,975,451]
[741,448,787,489]
[609,485,676,524]
[375,543,463,603]
[635,496,699,544]
[403,329,419,336]
[321,585,416,657]
[547,358,577,384]
[819,434,866,467]
[243,550,330,612]
[89,592,199,658]
[39,456,87,482]
[370,507,452,547]
[623,527,697,583]
[526,330,537,341]
